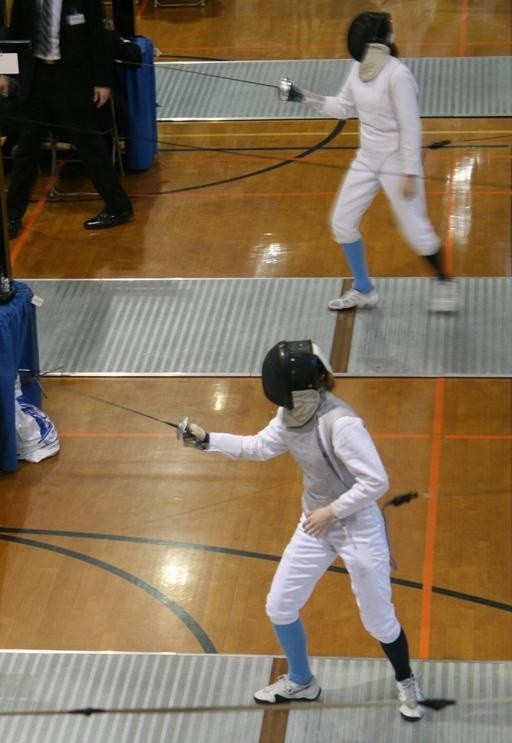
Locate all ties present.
[35,0,56,58]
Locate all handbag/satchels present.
[10,373,64,467]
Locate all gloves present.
[180,418,213,454]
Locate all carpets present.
[14,275,512,379]
[0,646,512,742]
[153,54,512,122]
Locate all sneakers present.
[323,284,383,312]
[393,672,428,722]
[252,669,324,706]
[427,275,462,315]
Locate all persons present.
[183,339,426,722]
[0,0,133,239]
[277,11,459,312]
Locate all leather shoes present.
[82,201,138,230]
[7,215,27,240]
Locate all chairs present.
[45,87,127,197]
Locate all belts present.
[32,55,64,65]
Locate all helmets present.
[257,338,335,410]
[342,4,396,63]
